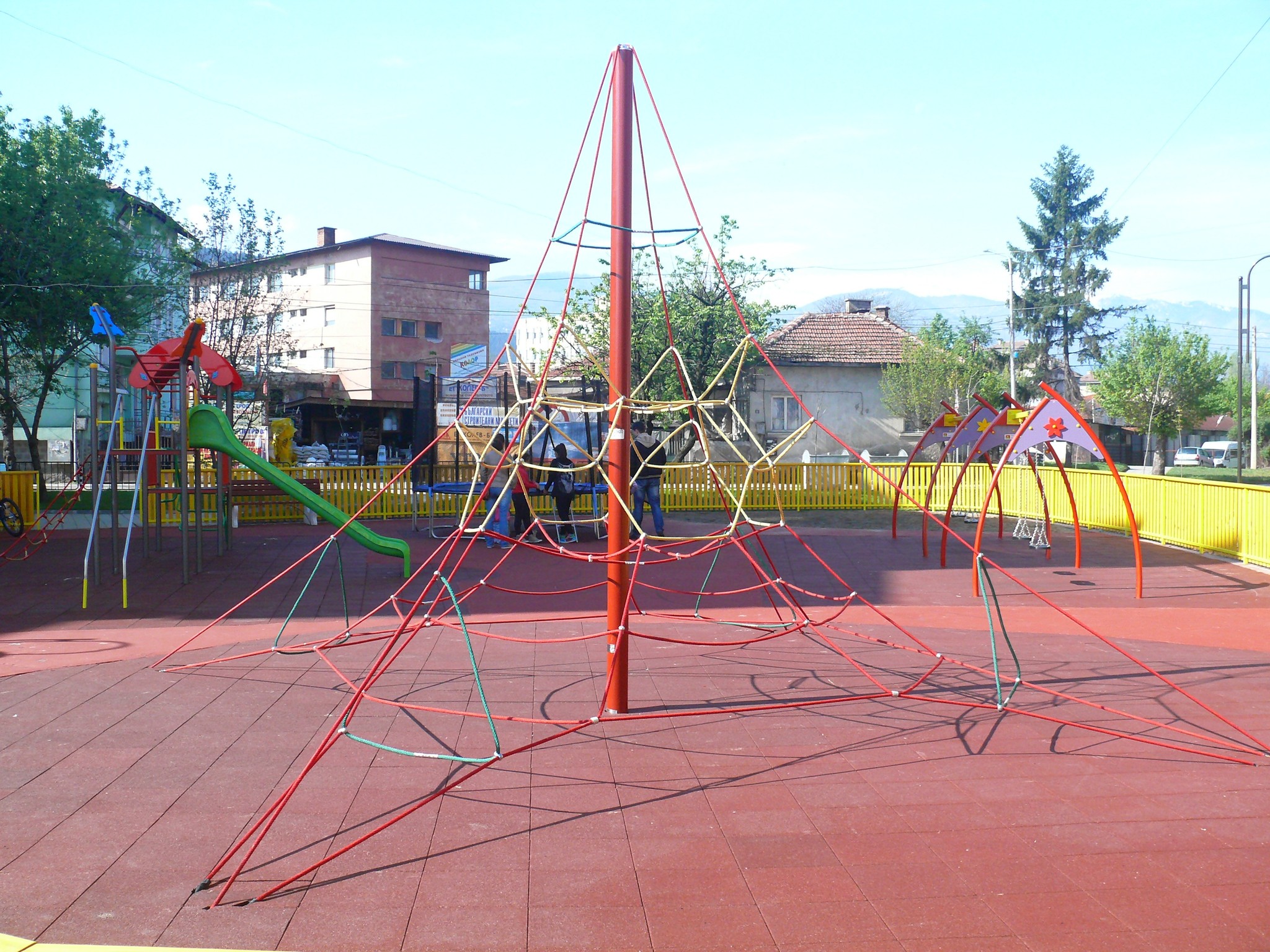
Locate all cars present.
[1174,446,1215,468]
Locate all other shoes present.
[629,532,639,540]
[501,543,513,548]
[658,532,664,536]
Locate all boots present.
[528,535,542,543]
[516,535,530,543]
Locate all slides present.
[188,406,411,577]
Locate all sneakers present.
[560,533,576,543]
[554,535,568,542]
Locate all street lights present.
[983,249,1017,409]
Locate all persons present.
[483,433,638,549]
[629,421,667,542]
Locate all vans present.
[1201,441,1246,467]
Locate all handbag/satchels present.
[481,489,489,501]
